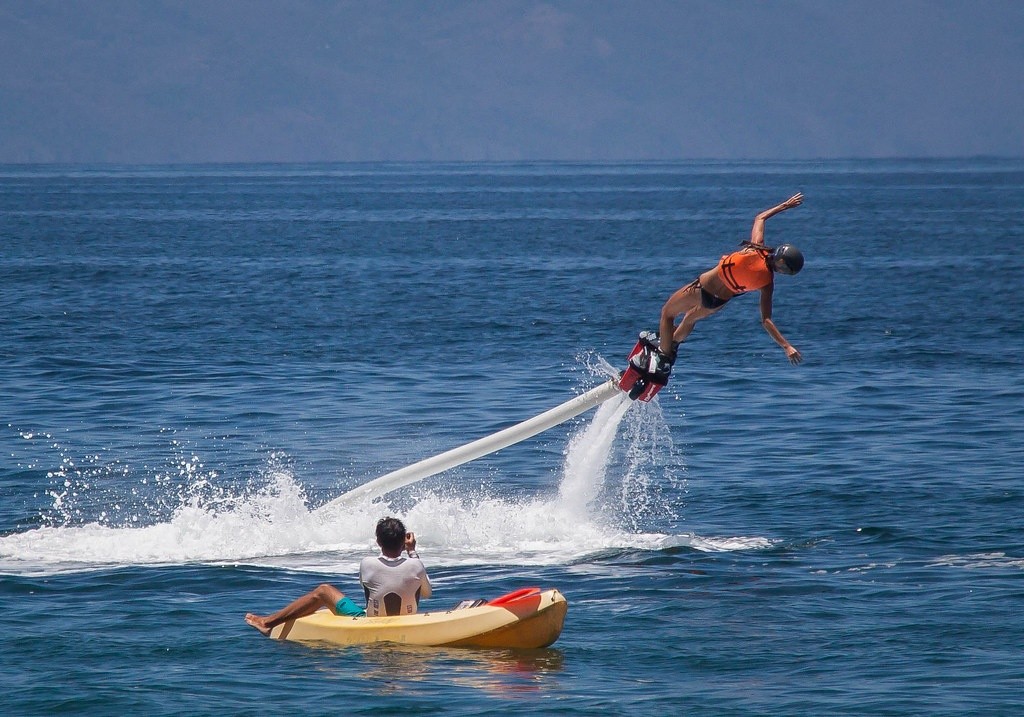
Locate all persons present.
[244,517,432,636]
[627,191,804,378]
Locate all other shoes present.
[634,331,679,374]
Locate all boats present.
[270,586,567,647]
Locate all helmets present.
[773,244,804,275]
